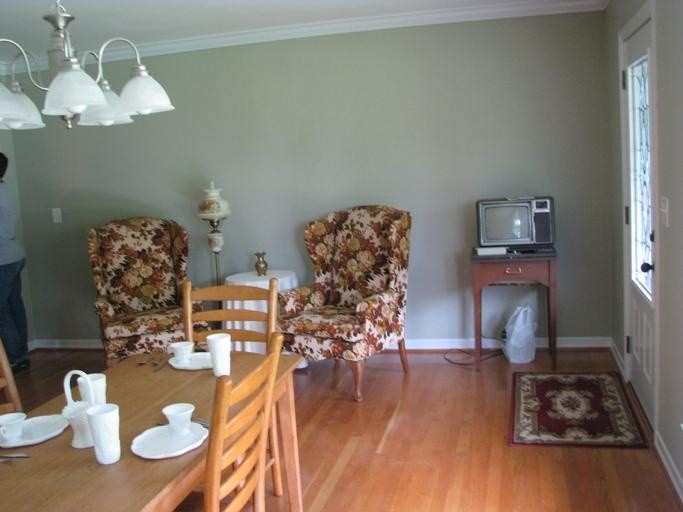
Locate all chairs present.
[184,278,279,496]
[0,349,24,418]
[174,332,284,511]
[276,206,411,404]
[88,217,210,369]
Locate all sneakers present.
[10,360,29,369]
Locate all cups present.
[206,332,232,378]
[0,412,28,441]
[85,403,121,465]
[161,401,195,435]
[76,373,107,406]
[170,341,194,365]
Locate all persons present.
[0,152,29,374]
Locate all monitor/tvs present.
[476,196,555,255]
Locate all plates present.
[0,413,70,449]
[168,351,212,371]
[130,421,209,460]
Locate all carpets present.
[510,368,647,450]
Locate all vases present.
[254,252,268,277]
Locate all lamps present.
[195,179,232,310]
[0,1,178,128]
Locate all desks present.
[470,247,559,372]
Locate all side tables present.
[224,269,305,369]
